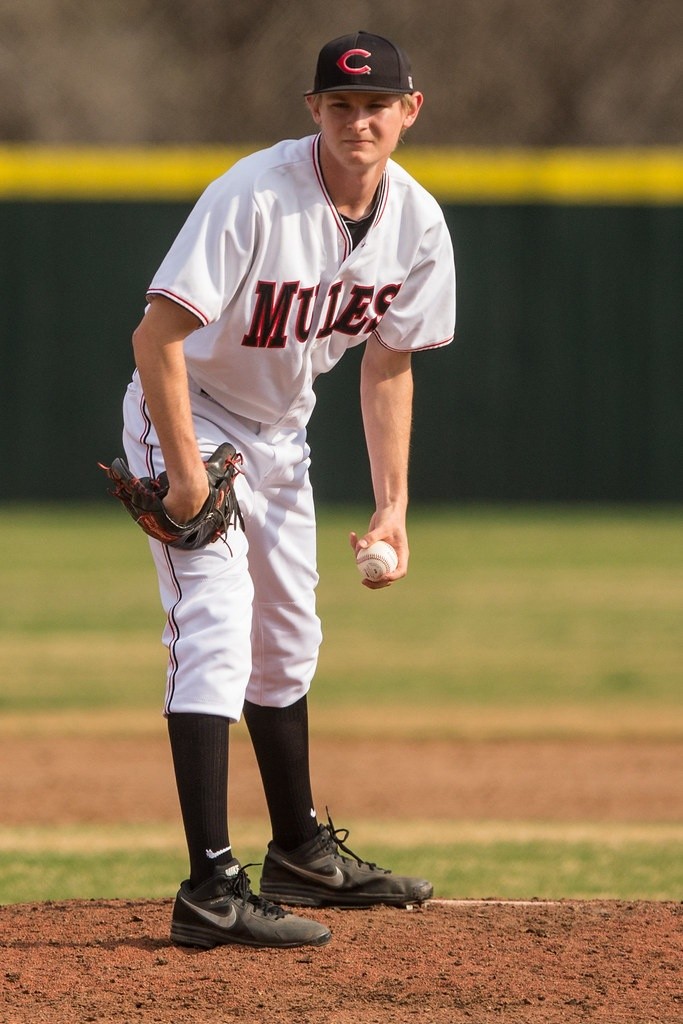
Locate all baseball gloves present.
[109,442,238,552]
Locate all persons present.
[101,31,459,947]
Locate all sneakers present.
[257,807,433,908]
[170,859,332,950]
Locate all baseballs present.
[355,540,399,582]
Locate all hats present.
[304,31,413,95]
